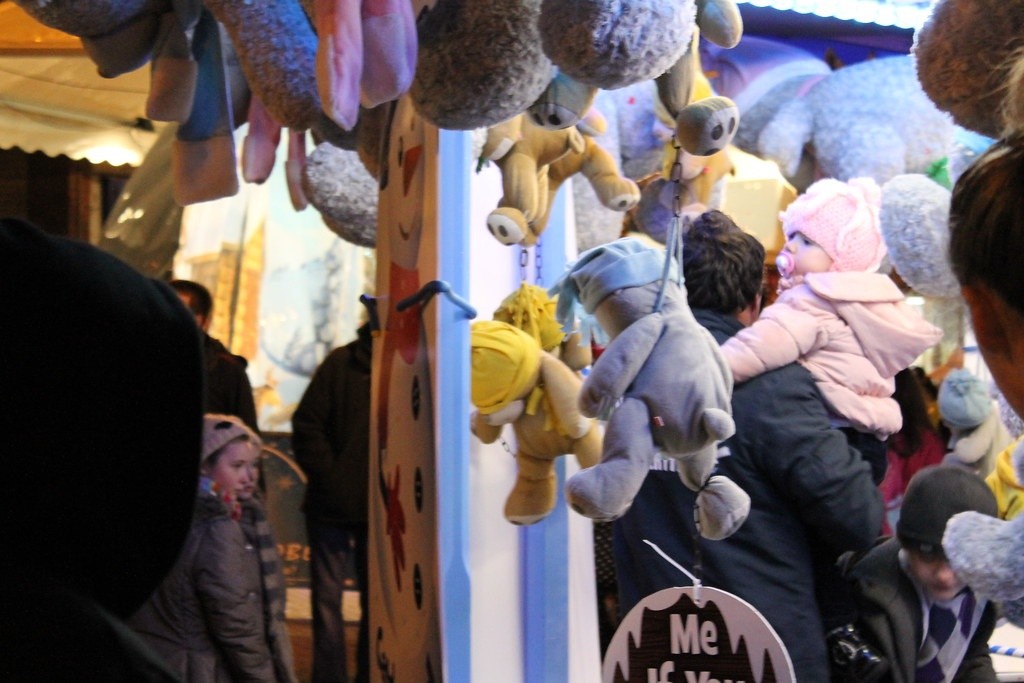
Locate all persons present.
[293,320,373,683]
[716,178,944,488]
[129,412,300,682]
[166,281,266,501]
[610,211,1023,683]
[0,218,205,683]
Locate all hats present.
[200,413,251,459]
[894,467,1000,563]
[1,210,205,613]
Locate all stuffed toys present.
[15,0,1023,628]
